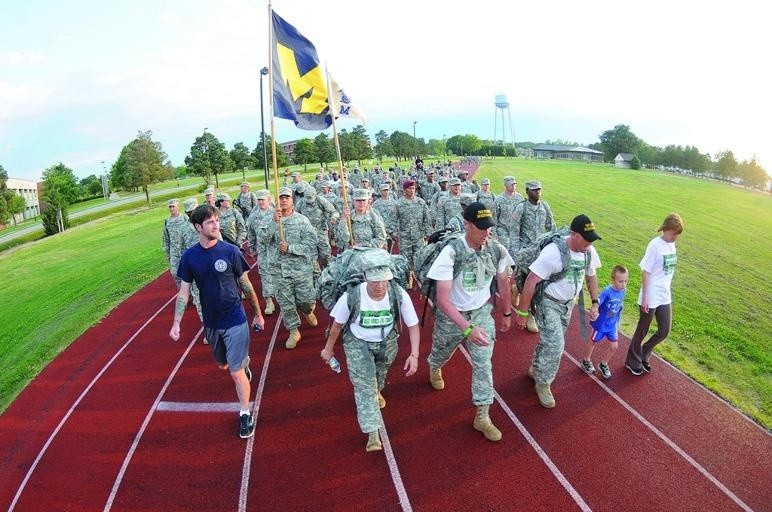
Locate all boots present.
[429,365,444,390]
[285,327,302,349]
[366,429,381,452]
[534,382,556,408]
[302,305,318,327]
[473,404,502,441]
[511,284,520,307]
[527,312,538,333]
[264,298,276,315]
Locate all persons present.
[581,265,628,379]
[169,205,264,439]
[514,213,602,409]
[161,154,556,349]
[319,250,421,453]
[426,202,516,442]
[624,214,683,375]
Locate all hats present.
[571,214,602,243]
[463,202,498,230]
[169,182,271,213]
[279,155,541,205]
[360,248,393,282]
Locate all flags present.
[271,10,367,131]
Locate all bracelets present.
[411,353,418,358]
[592,299,600,304]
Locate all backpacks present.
[413,232,501,310]
[515,226,591,307]
[315,246,410,312]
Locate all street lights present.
[443,134,447,158]
[259,63,271,192]
[412,120,417,140]
[203,128,210,153]
[100,161,114,198]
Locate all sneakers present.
[244,356,253,382]
[581,357,651,379]
[378,392,386,409]
[239,411,255,438]
